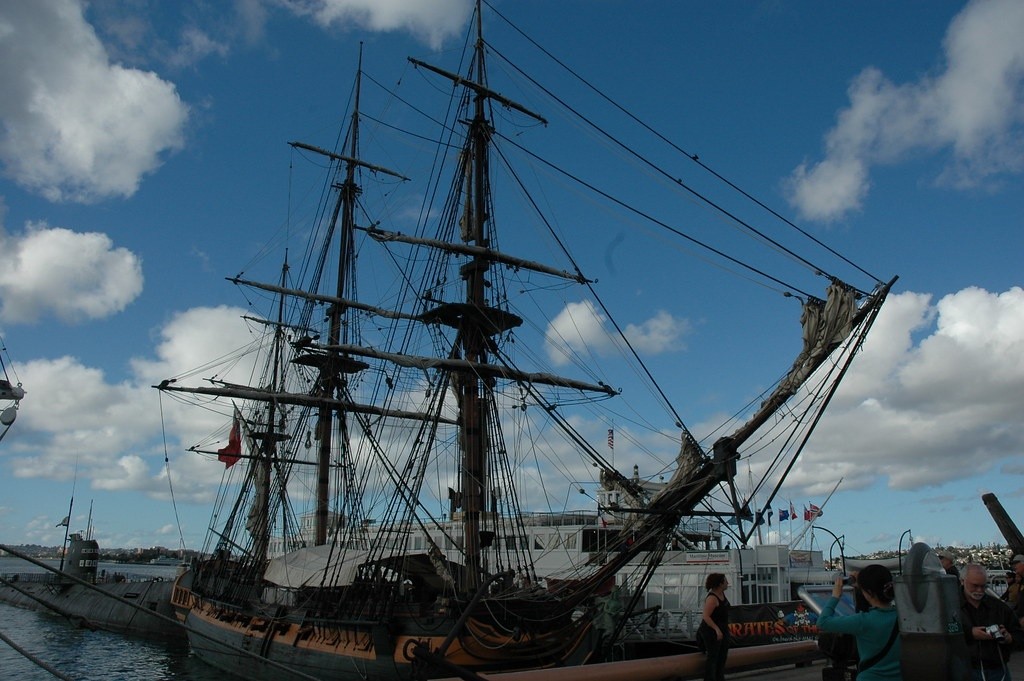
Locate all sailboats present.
[151,0,900,681]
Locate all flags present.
[768,502,773,526]
[608,429,613,449]
[779,509,789,521]
[56,516,69,527]
[805,503,823,522]
[218,408,241,469]
[790,502,798,520]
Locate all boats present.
[265,418,846,644]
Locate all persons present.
[725,541,731,550]
[816,564,900,681]
[937,551,1024,681]
[697,573,731,681]
[106,571,126,582]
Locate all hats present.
[938,552,954,562]
[1008,554,1024,565]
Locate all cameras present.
[985,624,1005,642]
[842,576,855,585]
